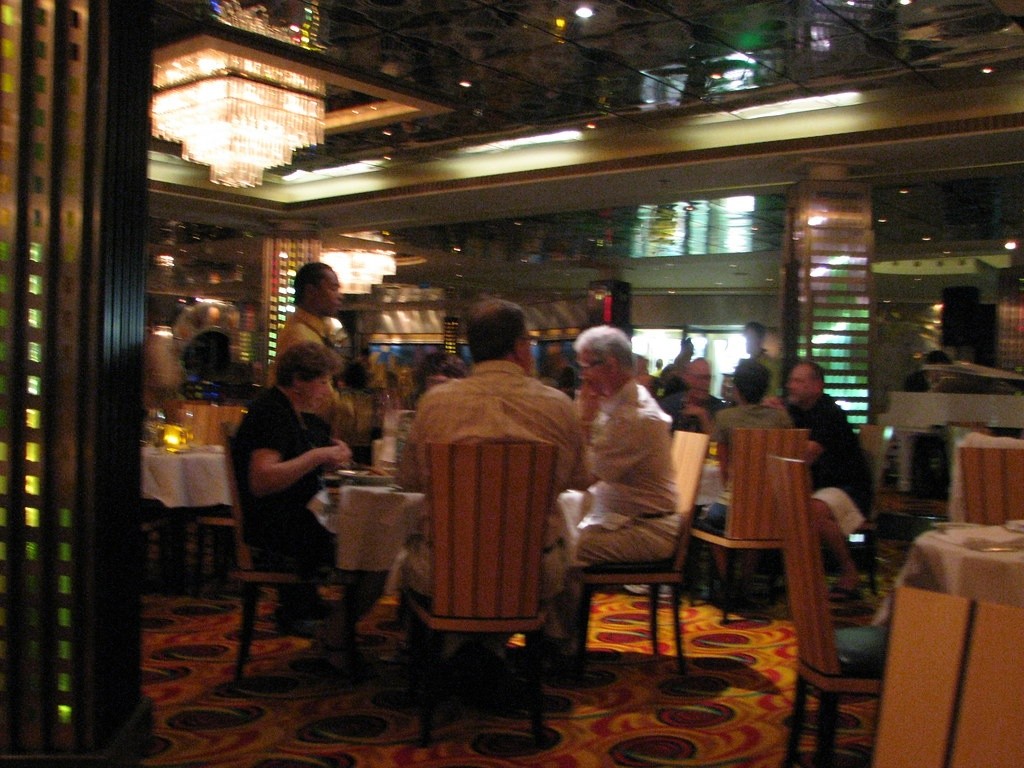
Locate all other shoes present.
[623,582,674,598]
[828,587,863,602]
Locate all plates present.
[930,522,987,535]
[335,469,398,486]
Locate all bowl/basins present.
[208,445,224,453]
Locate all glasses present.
[518,334,539,347]
[576,359,605,373]
[690,373,712,382]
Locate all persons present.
[506,325,680,675]
[397,297,584,697]
[231,342,389,659]
[779,363,874,599]
[896,351,967,497]
[686,363,791,607]
[142,261,783,440]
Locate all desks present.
[131,401,1024,712]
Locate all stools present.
[911,434,950,494]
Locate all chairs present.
[396,439,561,745]
[956,443,1024,526]
[765,454,891,768]
[165,398,250,601]
[690,427,812,622]
[568,429,712,675]
[822,424,894,588]
[870,585,1024,768]
[214,421,365,693]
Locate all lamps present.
[142,76,325,189]
